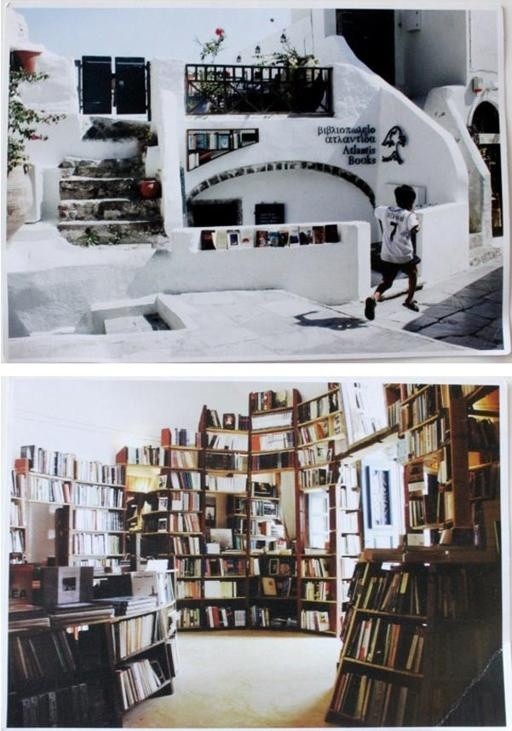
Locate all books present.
[9,636,176,727]
[10,385,499,637]
[329,637,501,726]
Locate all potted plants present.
[233,41,324,112]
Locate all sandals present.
[403,299,419,311]
[365,297,376,320]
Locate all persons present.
[364,184,423,320]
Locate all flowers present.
[188,28,230,113]
